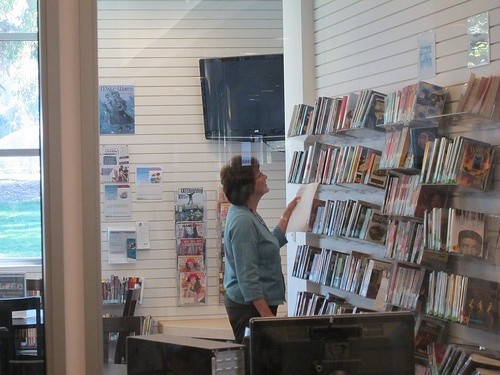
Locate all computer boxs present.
[126,334,247,375]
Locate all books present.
[14,290,42,347]
[98,84,233,336]
[272,76,500,375]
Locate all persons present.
[221,156,301,344]
[105,90,132,125]
[185,275,204,302]
[183,258,200,271]
[457,229,482,256]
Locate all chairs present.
[0,278,144,375]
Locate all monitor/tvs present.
[250,311,415,375]
[199,53,285,142]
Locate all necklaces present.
[244,204,263,222]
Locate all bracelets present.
[280,216,288,222]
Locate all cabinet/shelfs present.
[287,111,500,375]
